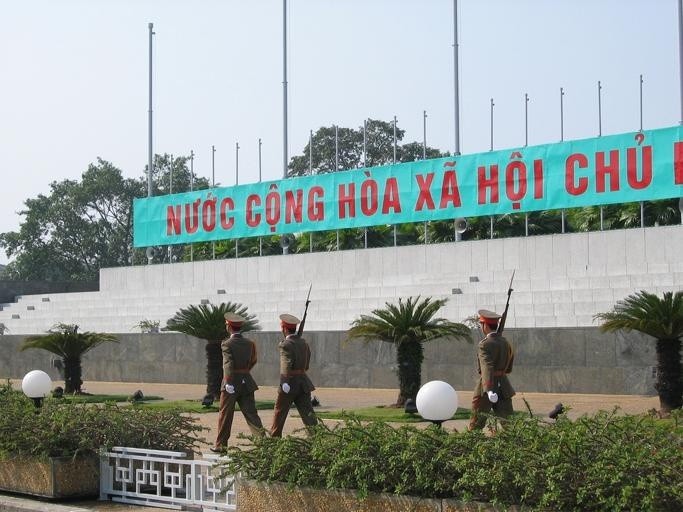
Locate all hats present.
[223,311,247,328]
[279,314,301,328]
[477,308,504,325]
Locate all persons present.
[270,313,318,438]
[467,309,515,432]
[210,312,267,454]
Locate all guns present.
[297,283,312,338]
[498,269,516,334]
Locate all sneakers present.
[209,446,229,454]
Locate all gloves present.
[487,391,499,404]
[281,383,291,393]
[225,384,237,394]
